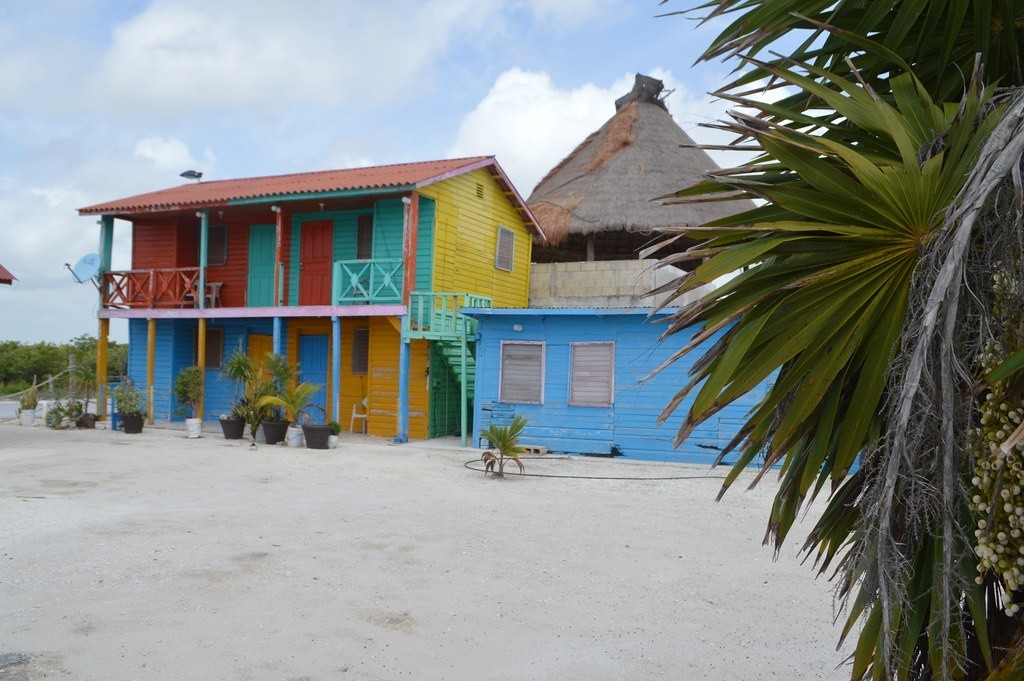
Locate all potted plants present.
[256,382,326,448]
[216,339,254,440]
[69,363,100,428]
[327,421,342,449]
[18,386,39,425]
[112,379,158,433]
[46,401,61,427]
[261,390,291,445]
[172,364,205,438]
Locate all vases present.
[303,424,332,448]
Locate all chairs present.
[348,396,367,435]
[351,276,369,305]
[181,278,206,308]
[205,281,223,307]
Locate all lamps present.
[179,169,203,184]
